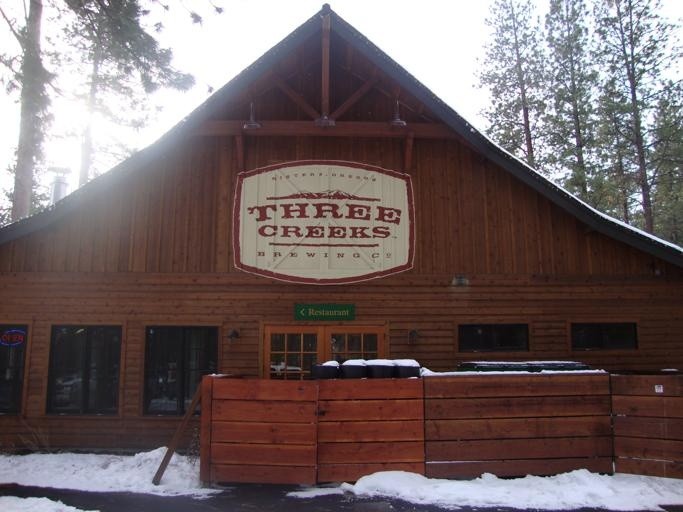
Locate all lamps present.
[242,102,261,129]
[314,113,336,128]
[410,329,417,337]
[391,100,407,126]
[227,330,241,339]
